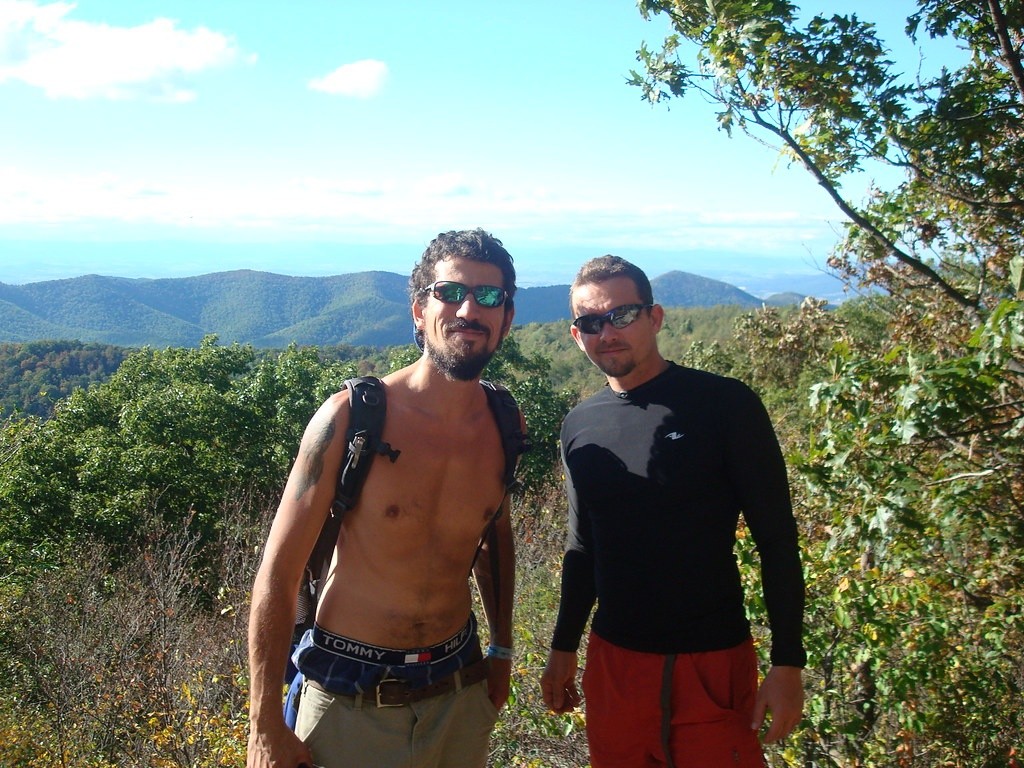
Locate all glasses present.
[573,304,654,334]
[424,281,508,307]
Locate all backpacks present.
[280,377,533,645]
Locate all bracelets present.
[488,646,513,660]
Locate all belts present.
[359,659,486,708]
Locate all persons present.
[538,254,807,768]
[246,229,528,768]
